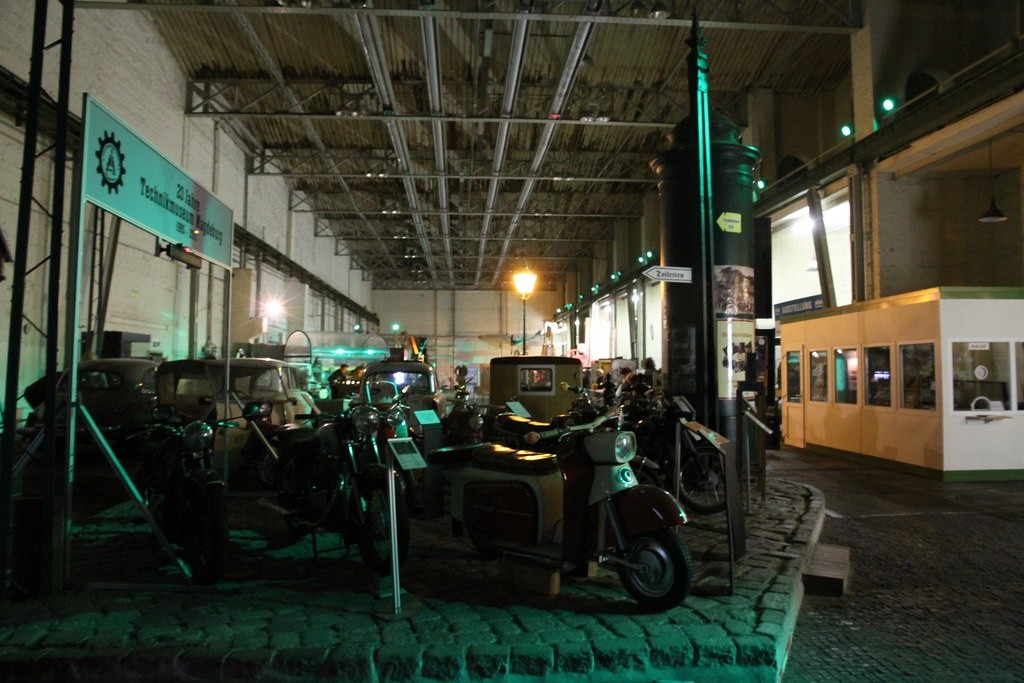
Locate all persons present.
[328,364,365,399]
[582,358,656,404]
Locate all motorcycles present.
[28,349,731,567]
[428,388,694,613]
[126,393,277,587]
